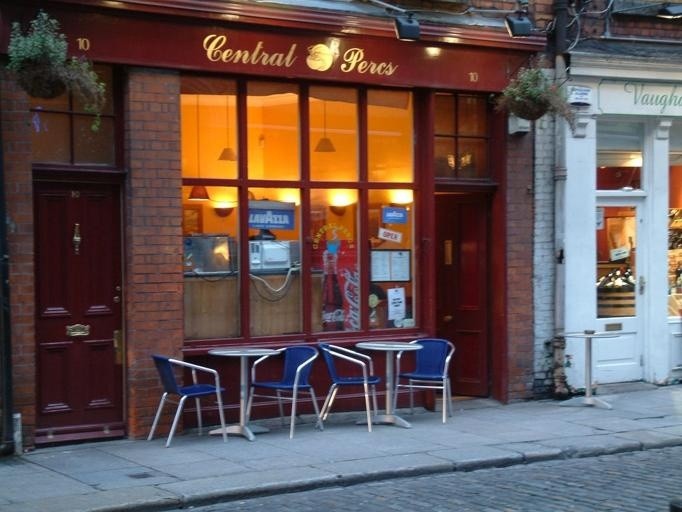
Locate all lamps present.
[395,12,423,41]
[328,204,347,215]
[185,92,237,218]
[505,15,536,42]
[314,101,337,156]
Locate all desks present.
[208,347,280,441]
[555,330,636,411]
[356,341,423,430]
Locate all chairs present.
[149,355,229,450]
[315,342,377,433]
[389,338,455,424]
[245,345,324,441]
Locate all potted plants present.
[7,10,109,139]
[490,54,581,139]
